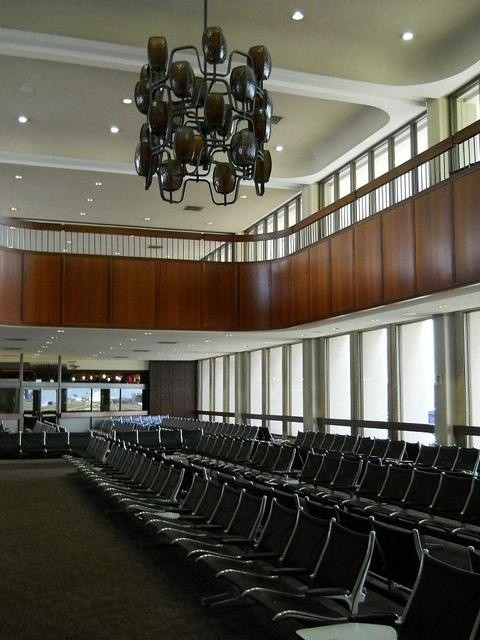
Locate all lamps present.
[134,0,272,209]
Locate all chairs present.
[218,507,336,596]
[359,437,378,457]
[316,435,336,451]
[436,448,461,469]
[144,477,227,535]
[376,440,390,458]
[88,445,137,480]
[241,518,377,622]
[233,443,253,467]
[157,482,246,544]
[284,454,324,490]
[95,413,157,436]
[101,457,155,493]
[119,465,185,509]
[300,458,343,496]
[213,441,243,472]
[70,436,97,460]
[79,441,120,470]
[348,461,390,508]
[73,432,87,447]
[66,438,93,457]
[256,448,282,479]
[193,498,302,578]
[21,431,45,455]
[368,464,414,515]
[158,415,267,450]
[427,444,442,462]
[175,491,269,556]
[395,469,445,522]
[328,460,363,503]
[432,473,475,527]
[395,440,406,456]
[46,429,69,457]
[133,473,209,524]
[346,434,360,455]
[93,450,149,486]
[102,461,165,497]
[451,477,480,538]
[1,432,21,454]
[138,430,161,450]
[283,430,307,449]
[27,419,66,438]
[114,430,137,446]
[268,448,298,483]
[83,442,126,475]
[240,443,269,477]
[404,441,423,463]
[303,431,317,450]
[462,448,480,471]
[70,442,110,467]
[333,436,346,452]
[69,437,101,464]
